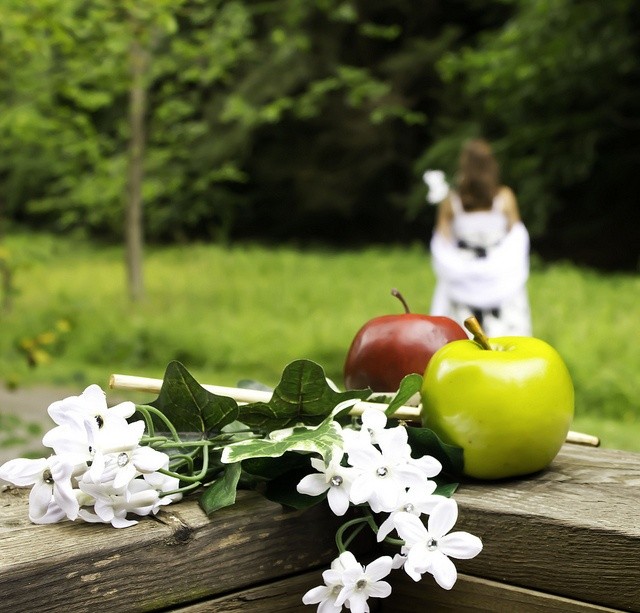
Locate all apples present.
[344,289,467,393]
[421,316,575,480]
